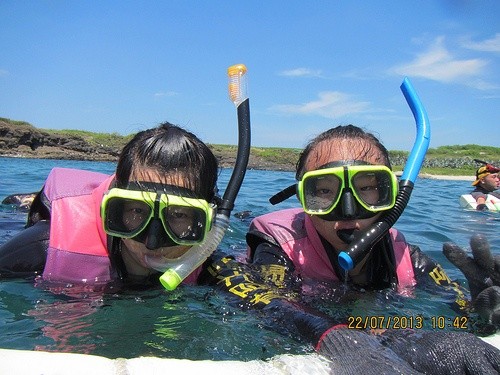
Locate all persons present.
[458,160,500,213]
[232,123,500,374]
[0,122,425,375]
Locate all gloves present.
[441,233,500,335]
[317,328,427,375]
[376,323,500,375]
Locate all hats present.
[472,166,498,186]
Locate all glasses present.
[292,161,399,221]
[99,183,217,248]
